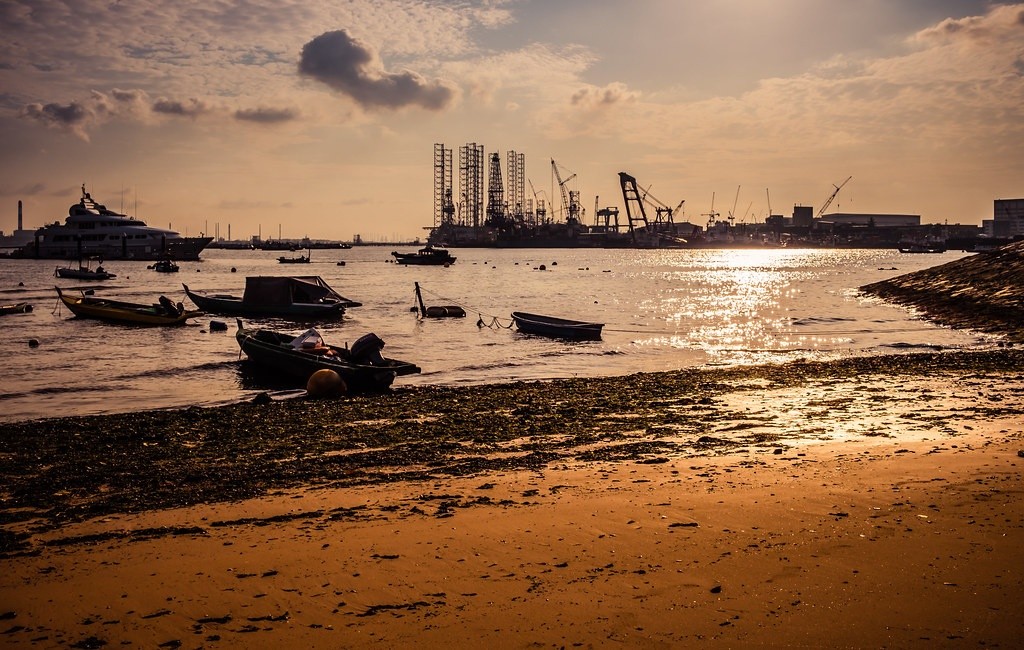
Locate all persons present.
[157,295,180,316]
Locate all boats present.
[276,256,309,263]
[56,287,204,326]
[305,241,353,249]
[427,305,467,318]
[181,276,364,321]
[260,241,303,251]
[10,184,215,261]
[152,234,180,272]
[391,245,457,265]
[964,236,1006,252]
[236,318,422,394]
[511,311,606,342]
[897,235,947,254]
[57,266,116,280]
